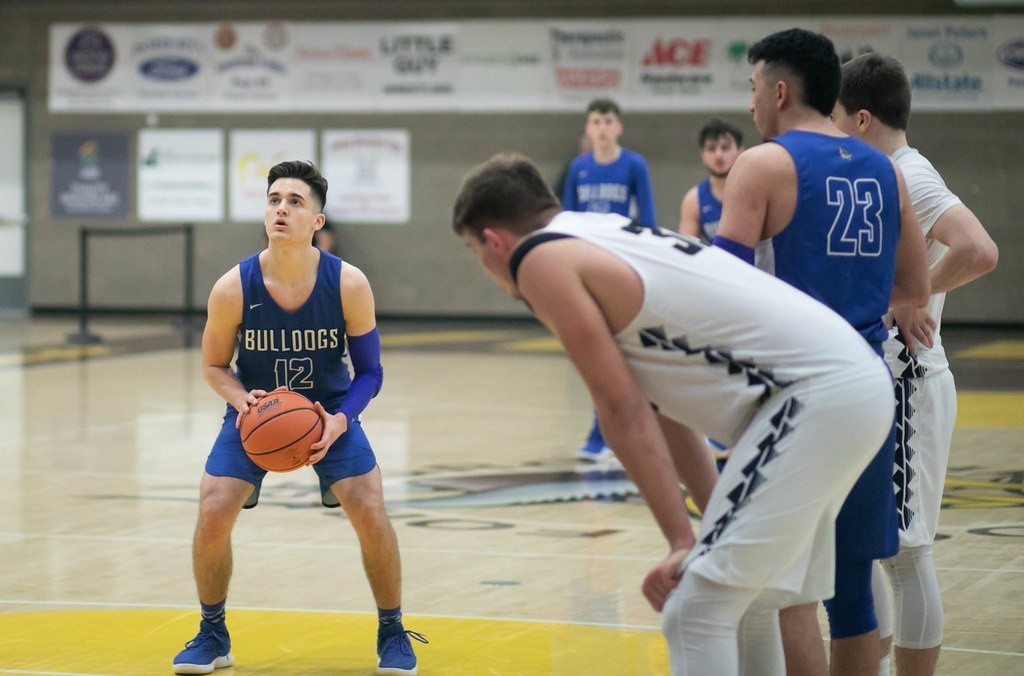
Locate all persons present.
[558,95,751,515]
[831,49,1001,675]
[305,211,336,254]
[448,151,900,676]
[706,27,933,675]
[169,157,419,675]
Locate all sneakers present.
[172,621,235,673]
[375,622,418,675]
[576,432,611,463]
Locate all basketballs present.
[239,390,327,473]
